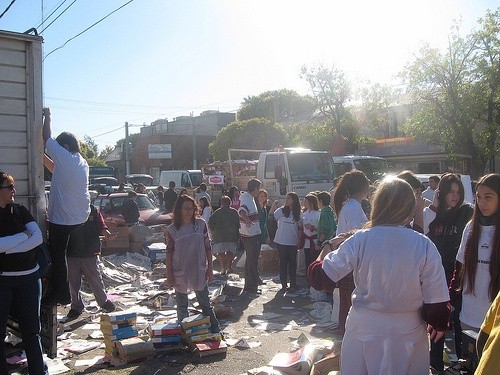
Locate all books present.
[99,310,227,366]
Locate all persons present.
[118,179,337,334]
[0,171,49,375]
[43,108,91,298]
[67,204,116,318]
[307,171,500,375]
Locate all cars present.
[90,173,174,228]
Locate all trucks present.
[329,155,392,183]
[160,170,202,196]
[227,146,336,208]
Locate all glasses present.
[1,183,15,190]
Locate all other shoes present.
[429,366,447,375]
[453,353,473,374]
[328,325,345,332]
[67,308,82,320]
[100,300,115,312]
